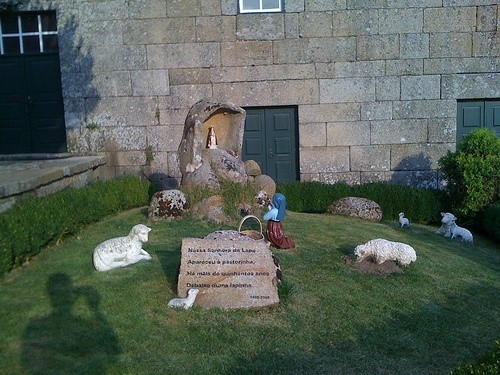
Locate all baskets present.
[239,215,264,241]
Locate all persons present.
[207,126,218,149]
[262,193,295,249]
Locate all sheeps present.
[353,237,417,266]
[440,212,474,245]
[399,212,410,228]
[166,288,200,310]
[93,223,152,272]
